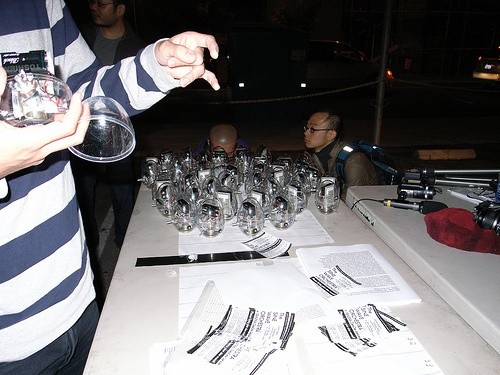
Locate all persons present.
[0,0,221,375]
[68,0,147,248]
[192,124,249,163]
[294,110,379,202]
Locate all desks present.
[347,186,500,357]
[83,183,500,375]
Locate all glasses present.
[304,125,337,133]
[88,0,121,8]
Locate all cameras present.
[394,166,437,199]
[472,202,500,237]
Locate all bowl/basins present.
[0,73,72,127]
[68,96,136,163]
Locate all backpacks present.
[336,138,401,198]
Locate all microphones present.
[380,198,448,214]
